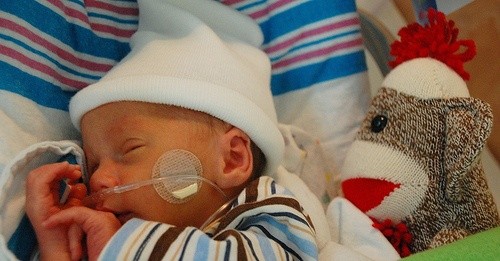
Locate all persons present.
[26,0,320,261]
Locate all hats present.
[66,0,286,177]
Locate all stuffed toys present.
[339,7,500,258]
